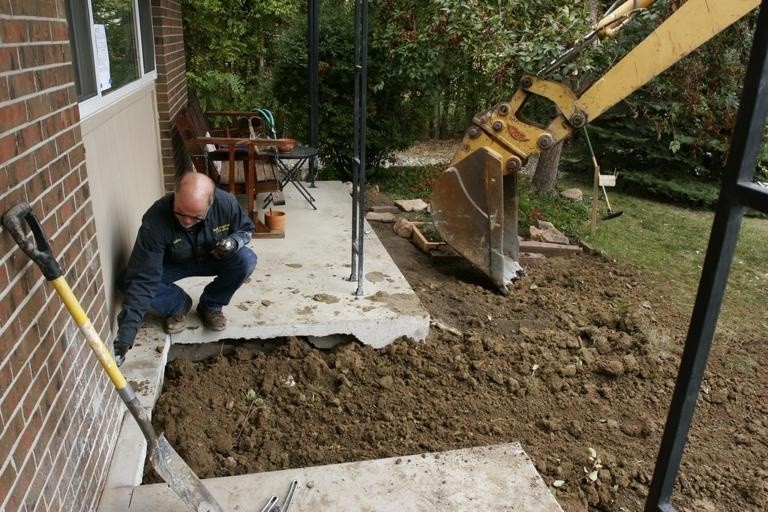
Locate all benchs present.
[176,87,296,239]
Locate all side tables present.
[255,144,318,209]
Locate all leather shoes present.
[164,291,193,333]
[197,303,226,331]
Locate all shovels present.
[3,201,224,512]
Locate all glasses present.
[175,211,208,221]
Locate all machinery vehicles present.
[429,0,767,297]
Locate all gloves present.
[114,341,130,367]
[214,238,237,259]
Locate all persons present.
[113,171,257,367]
